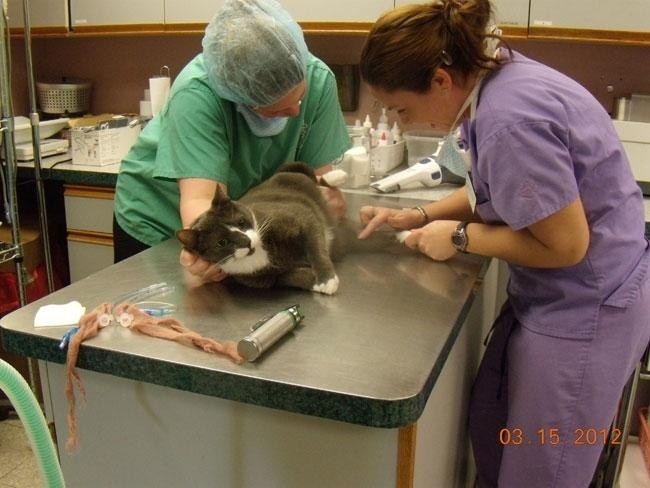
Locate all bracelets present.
[412,205,432,226]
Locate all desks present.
[1,136,126,189]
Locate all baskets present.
[36,79,92,115]
[0,226,44,275]
[70,124,142,168]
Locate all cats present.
[175,161,425,297]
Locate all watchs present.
[449,220,474,254]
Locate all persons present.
[113,1,352,290]
[358,0,650,486]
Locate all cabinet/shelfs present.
[3,0,165,38]
[165,0,394,38]
[61,182,115,282]
[486,0,650,45]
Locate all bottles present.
[354,107,402,148]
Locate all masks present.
[236,105,289,138]
[435,134,473,179]
